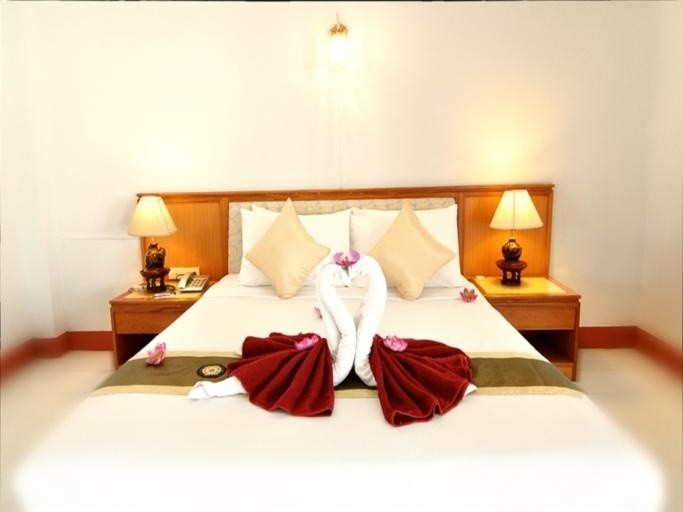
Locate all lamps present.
[489,188,544,286]
[128,196,178,293]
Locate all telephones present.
[178,270,210,292]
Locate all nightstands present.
[108,280,218,371]
[468,274,582,382]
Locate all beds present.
[1,191,664,512]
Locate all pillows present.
[237,197,465,300]
